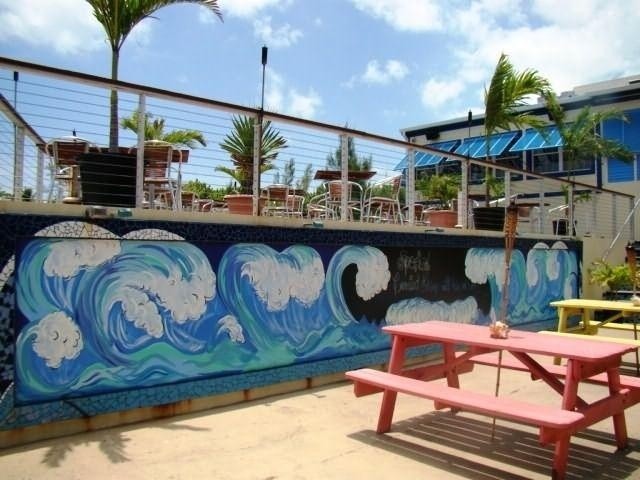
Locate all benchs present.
[538,329,639,373]
[346,367,585,479]
[578,319,639,331]
[468,352,639,447]
[343,351,640,480]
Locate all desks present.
[553,299,639,332]
[381,319,639,427]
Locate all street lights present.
[14,72,18,109]
[469,112,472,137]
[262,47,267,110]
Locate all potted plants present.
[471,51,636,235]
[412,172,465,229]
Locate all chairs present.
[46,137,99,203]
[180,169,423,224]
[129,138,182,209]
